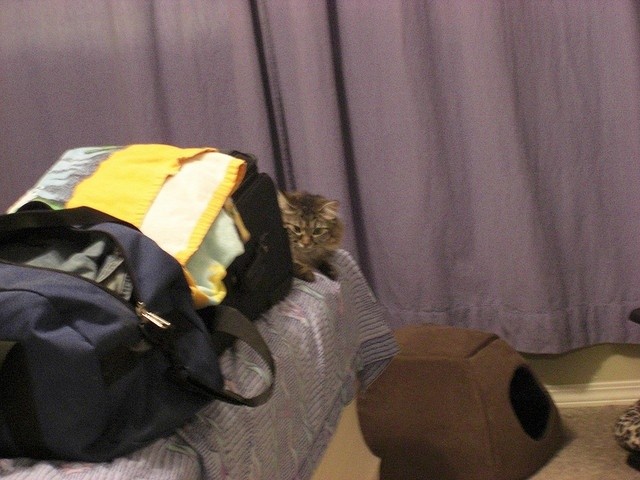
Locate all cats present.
[277,187,347,283]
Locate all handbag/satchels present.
[197,149,293,359]
[0,200,277,464]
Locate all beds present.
[0,248,402,479]
